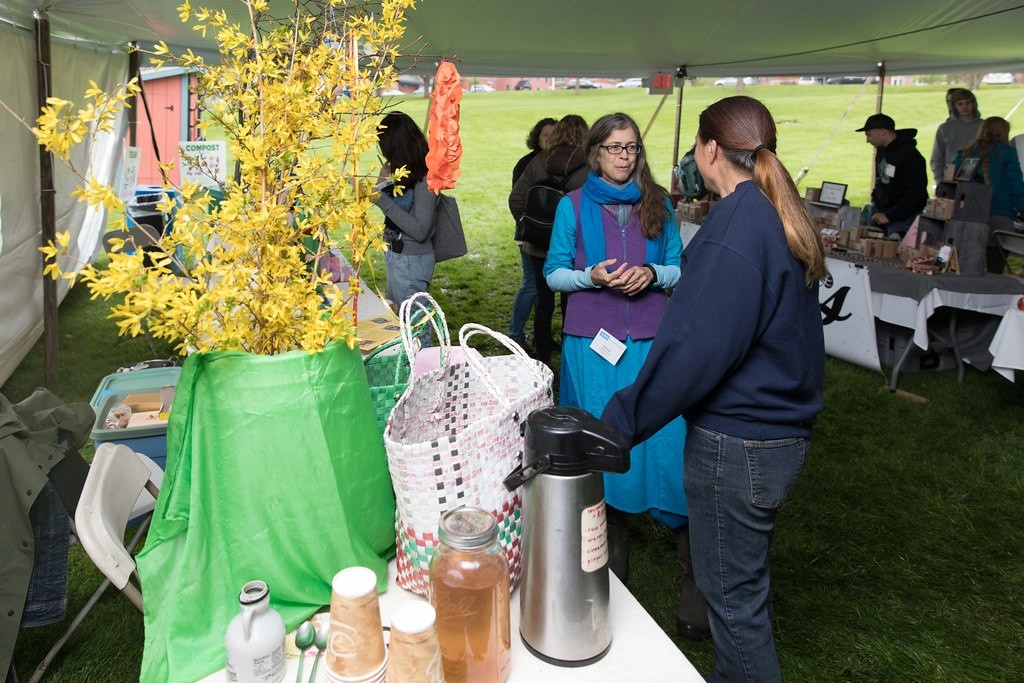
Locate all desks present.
[676,220,1024,392]
[806,200,841,218]
[321,243,422,356]
[195,556,709,683]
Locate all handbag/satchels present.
[431,193,467,263]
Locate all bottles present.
[429,506,512,683]
[936,237,954,274]
[225,581,286,683]
[951,149,964,179]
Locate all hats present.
[855,113,895,130]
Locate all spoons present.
[294,619,328,683]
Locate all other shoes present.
[509,333,532,355]
[606,519,630,585]
[533,333,562,351]
[677,618,712,641]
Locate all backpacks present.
[514,163,587,249]
[672,154,708,202]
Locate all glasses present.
[598,144,642,154]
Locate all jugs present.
[836,206,862,232]
[503,407,632,667]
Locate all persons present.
[598,97,828,683]
[952,116,1024,276]
[854,113,930,239]
[929,88,990,202]
[361,111,437,349]
[681,132,711,202]
[507,114,595,357]
[511,117,566,353]
[541,111,688,582]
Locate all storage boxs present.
[927,197,954,220]
[944,164,956,180]
[805,187,820,202]
[875,320,957,374]
[90,366,182,471]
[838,225,897,258]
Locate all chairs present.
[1,392,164,682]
[74,442,161,616]
[101,223,197,358]
[992,230,1024,274]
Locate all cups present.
[942,165,954,182]
[924,197,954,220]
[386,600,446,683]
[322,567,387,683]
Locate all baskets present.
[363,307,445,490]
[383,292,555,599]
